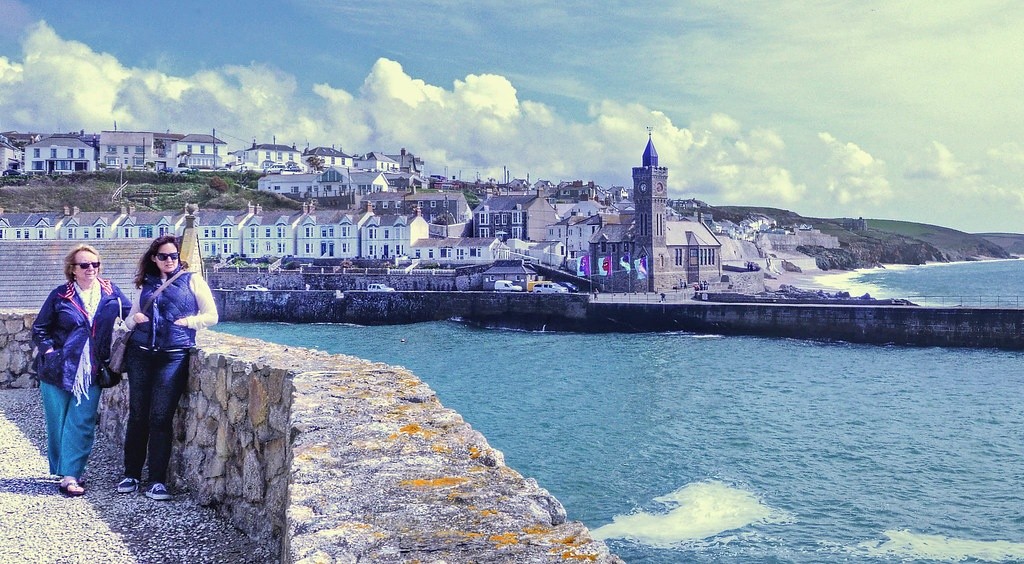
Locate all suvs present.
[367,284,394,292]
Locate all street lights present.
[549,242,554,269]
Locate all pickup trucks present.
[533,283,568,294]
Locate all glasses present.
[70,262,100,269]
[153,252,180,261]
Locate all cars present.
[191,167,200,172]
[3,170,21,177]
[559,282,579,293]
[290,166,302,172]
[157,167,173,174]
[246,284,268,292]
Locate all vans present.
[263,164,286,175]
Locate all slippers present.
[60,481,85,495]
[60,478,85,486]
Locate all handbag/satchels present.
[99,361,121,388]
[107,337,126,373]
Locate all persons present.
[693,280,707,291]
[594,287,599,297]
[119,236,218,499]
[660,293,666,301]
[747,261,753,272]
[32,244,132,495]
[684,280,687,289]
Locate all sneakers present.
[118,477,140,493]
[145,481,171,500]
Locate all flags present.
[577,256,590,276]
[598,256,611,275]
[620,254,630,273]
[634,256,647,279]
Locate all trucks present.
[495,281,522,293]
